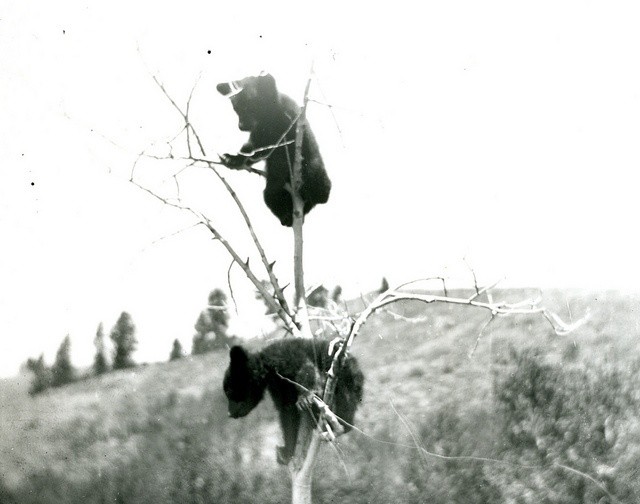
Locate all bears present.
[222,337,366,466]
[216,73,332,227]
